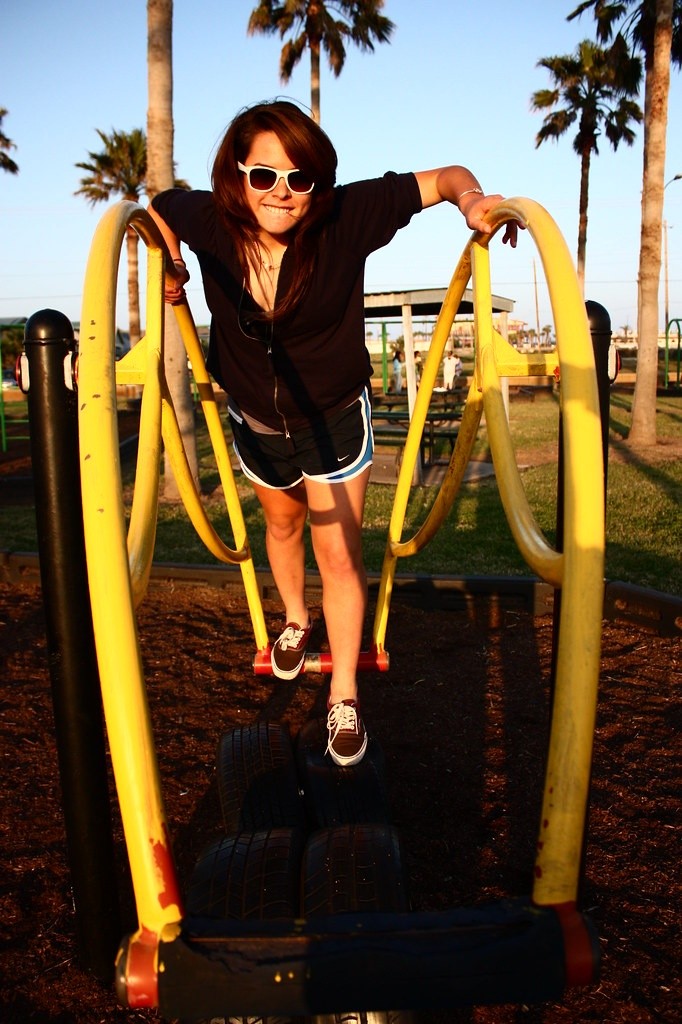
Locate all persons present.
[146,102,519,767]
[414,351,423,389]
[442,349,463,389]
[392,351,404,394]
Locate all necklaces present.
[252,241,280,269]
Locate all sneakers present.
[271,614,313,680]
[323,691,367,766]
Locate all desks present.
[385,386,473,411]
[370,412,462,465]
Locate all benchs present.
[383,400,466,411]
[372,429,458,468]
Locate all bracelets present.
[173,259,186,267]
[458,187,484,200]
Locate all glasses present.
[237,161,314,194]
